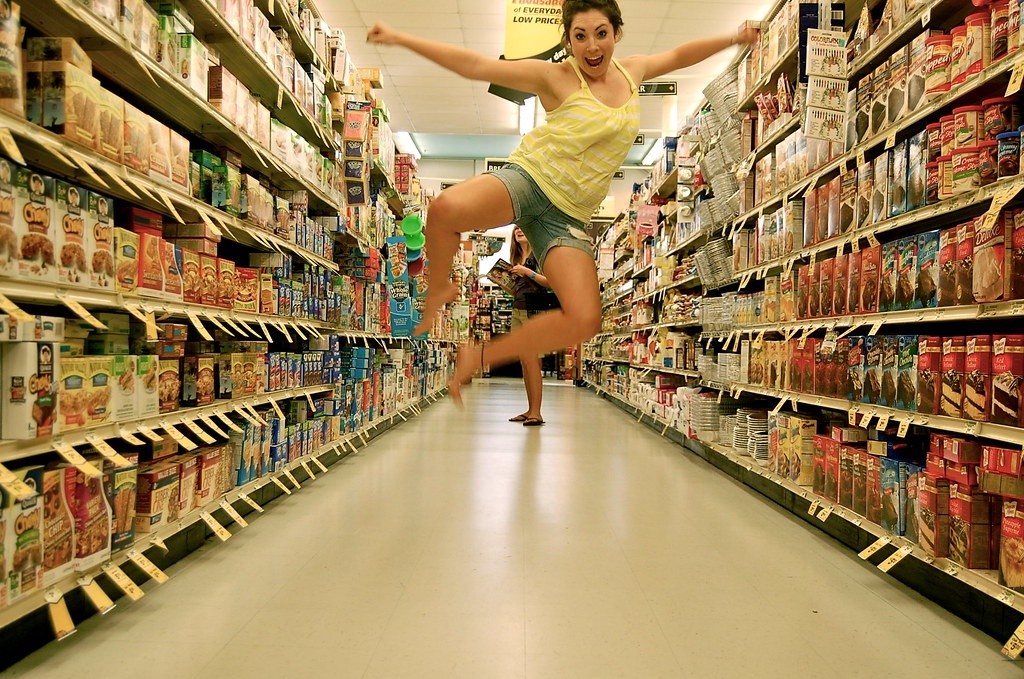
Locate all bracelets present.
[531,272,536,280]
[731,37,734,45]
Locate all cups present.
[638,223,653,249]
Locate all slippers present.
[508,414,527,421]
[523,418,546,425]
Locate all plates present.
[403,231,425,250]
[409,257,424,276]
[401,216,422,236]
[407,248,422,261]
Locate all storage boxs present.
[1,0,453,624]
[735,1,1024,592]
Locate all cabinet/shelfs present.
[479,282,511,334]
[569,0,1024,620]
[1,0,478,647]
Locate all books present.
[486,259,518,296]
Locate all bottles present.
[737,292,763,324]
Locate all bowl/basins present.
[727,409,768,467]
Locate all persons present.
[489,268,510,281]
[510,223,553,426]
[369,0,758,406]
